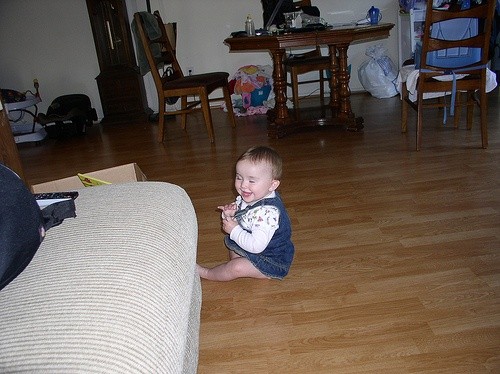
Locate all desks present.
[224,23,395,138]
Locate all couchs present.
[0,182,202,374]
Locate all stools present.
[285,56,340,110]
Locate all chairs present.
[134,10,236,145]
[401,0,498,152]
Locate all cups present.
[284,11,302,29]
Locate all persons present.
[196,144,295,281]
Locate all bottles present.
[245,15,255,37]
[366,6,383,27]
